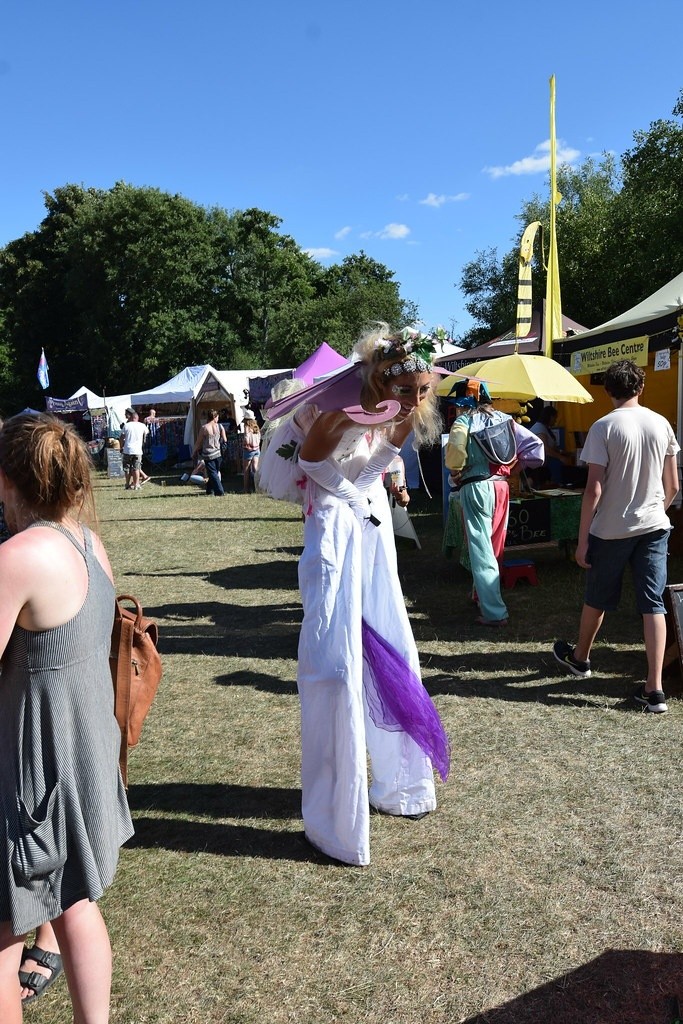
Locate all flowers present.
[374,324,453,363]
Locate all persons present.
[529,406,573,468]
[0,411,136,1024]
[443,384,545,626]
[550,359,680,713]
[190,409,227,496]
[264,326,441,679]
[241,409,262,493]
[118,408,150,490]
[144,409,159,426]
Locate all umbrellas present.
[437,351,595,404]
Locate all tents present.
[390,271,683,490]
[47,327,465,474]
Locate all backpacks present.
[109,595,162,791]
[466,409,518,464]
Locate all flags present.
[37,352,49,388]
[515,220,541,337]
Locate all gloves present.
[447,473,462,487]
[298,453,370,532]
[353,437,401,493]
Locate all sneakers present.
[554,641,592,677]
[631,682,668,712]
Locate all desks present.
[441,488,585,574]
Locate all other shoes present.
[134,486,144,490]
[472,615,508,627]
[140,476,151,485]
[125,484,132,490]
[465,591,480,609]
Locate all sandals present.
[18,944,63,1003]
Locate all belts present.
[449,475,507,492]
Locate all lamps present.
[566,330,576,337]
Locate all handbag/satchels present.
[219,423,227,454]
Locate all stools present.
[502,558,538,589]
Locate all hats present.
[243,410,256,420]
[446,378,493,408]
